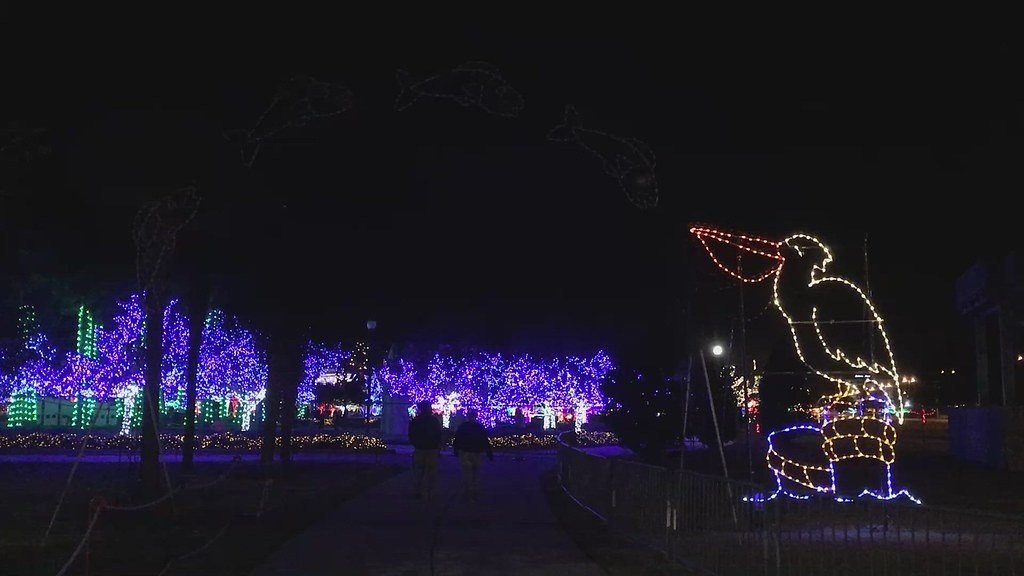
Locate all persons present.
[453,408,495,503]
[408,400,442,502]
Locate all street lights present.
[366,320,378,437]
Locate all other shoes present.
[415,493,425,499]
